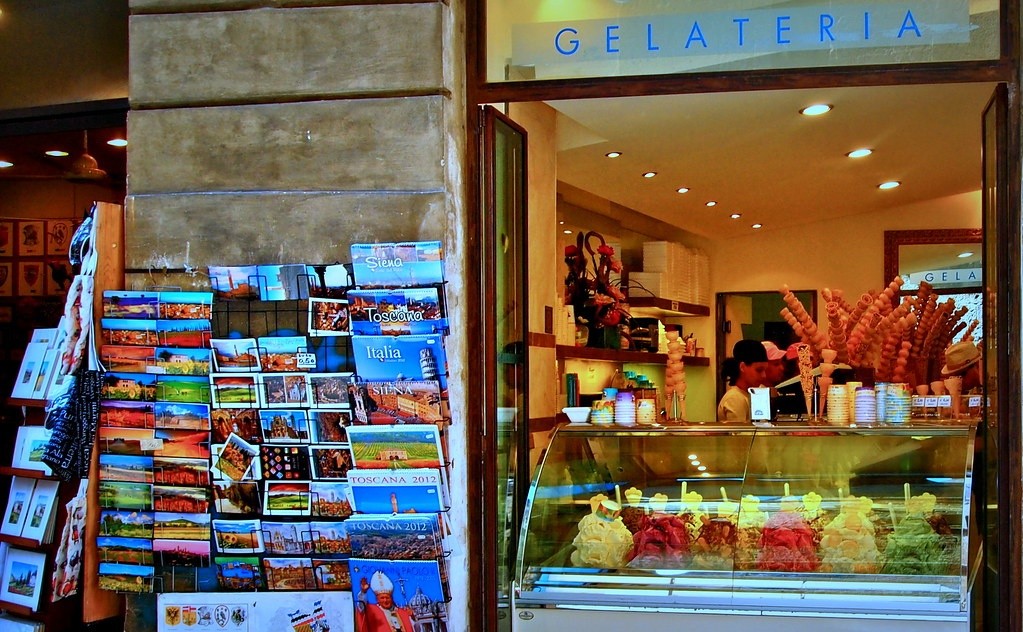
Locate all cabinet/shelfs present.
[0,202,453,632]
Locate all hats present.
[760,341,787,361]
[733,339,768,362]
[785,342,814,361]
[370,571,394,595]
[941,341,983,374]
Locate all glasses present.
[957,363,975,377]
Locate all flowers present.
[566,230,656,349]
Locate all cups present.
[615,392,637,427]
[854,387,877,423]
[596,500,621,522]
[827,385,849,422]
[638,399,656,425]
[602,388,618,400]
[847,379,862,423]
[591,400,614,426]
[887,383,911,422]
[874,382,889,421]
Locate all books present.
[97,246,453,632]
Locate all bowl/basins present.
[562,407,591,423]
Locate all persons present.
[941,341,984,452]
[354,577,421,632]
[785,343,815,380]
[758,341,785,421]
[716,339,767,423]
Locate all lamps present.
[64,132,107,182]
